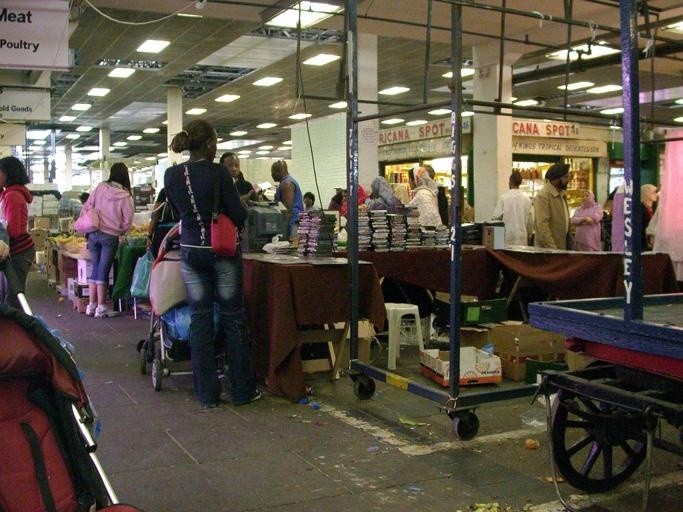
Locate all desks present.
[335,243,487,298]
[114,245,154,321]
[241,253,388,402]
[485,244,674,350]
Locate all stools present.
[371,302,425,372]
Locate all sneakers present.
[250,389,262,403]
[86,305,120,318]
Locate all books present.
[358,211,449,250]
[296,211,336,258]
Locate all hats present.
[545,163,570,181]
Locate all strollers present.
[138,222,221,392]
[0,259,146,511]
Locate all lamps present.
[260,0,346,32]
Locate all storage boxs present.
[420,347,501,388]
[492,324,567,382]
[24,194,115,316]
[431,297,508,326]
[326,320,373,368]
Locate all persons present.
[217,151,448,228]
[640,183,657,253]
[78,163,134,318]
[572,190,604,252]
[0,155,35,309]
[163,120,262,410]
[534,163,571,251]
[145,186,180,259]
[490,172,533,247]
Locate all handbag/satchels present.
[74,209,100,233]
[158,202,176,223]
[211,212,238,257]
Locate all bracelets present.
[146,233,151,240]
